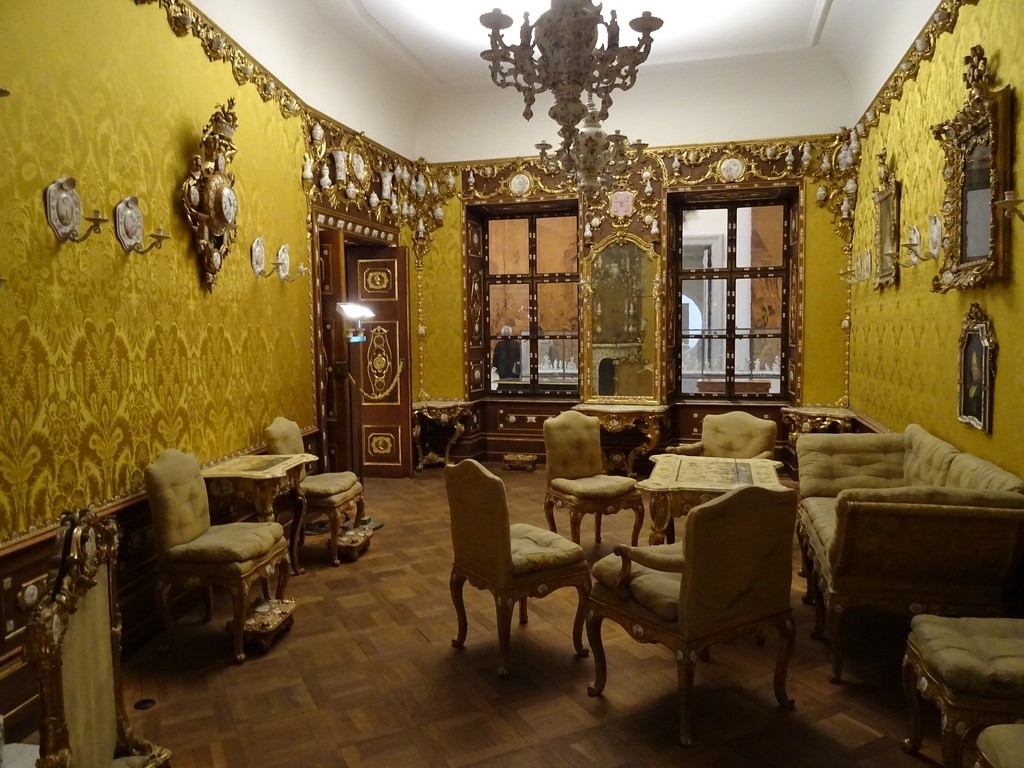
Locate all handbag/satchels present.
[512,360,521,374]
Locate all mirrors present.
[580,219,662,407]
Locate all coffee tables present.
[636,453,794,545]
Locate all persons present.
[492,326,521,378]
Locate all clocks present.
[183,97,241,292]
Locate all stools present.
[902,614,1023,767]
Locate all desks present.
[201,454,318,549]
[572,404,669,479]
[781,407,856,479]
[412,401,475,470]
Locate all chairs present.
[147,448,292,662]
[266,416,366,566]
[542,410,644,548]
[585,485,795,743]
[444,460,589,675]
[665,411,776,460]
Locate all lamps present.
[337,299,375,344]
[480,0,666,206]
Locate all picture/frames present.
[933,46,1014,292]
[958,301,996,433]
[874,150,900,289]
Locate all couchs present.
[796,425,1024,685]
